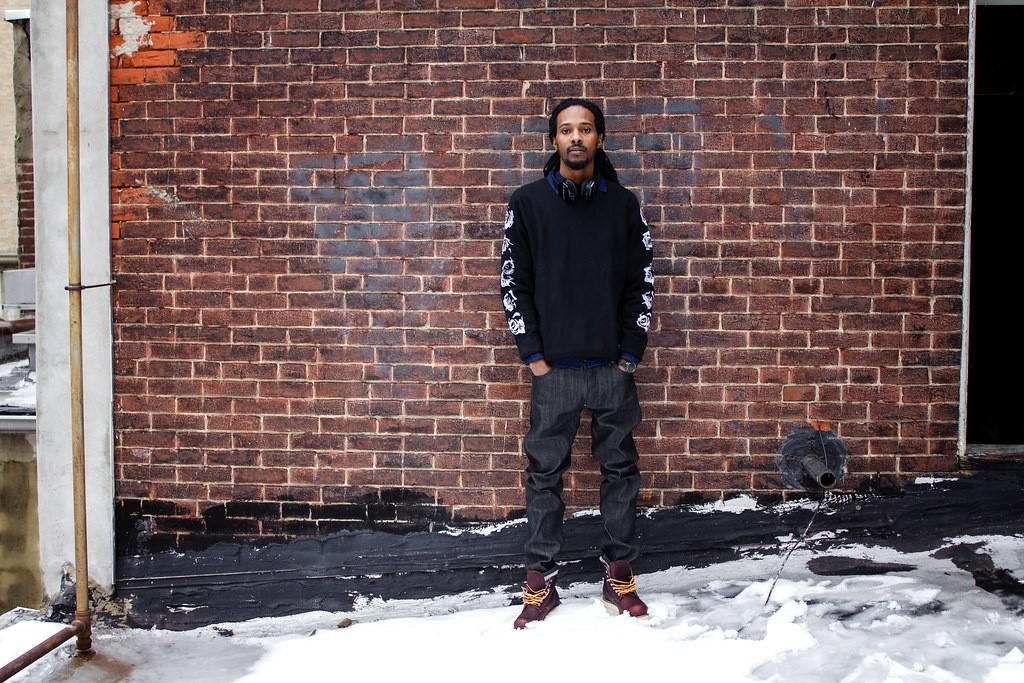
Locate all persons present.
[500,97,654,629]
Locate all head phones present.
[550,165,597,204]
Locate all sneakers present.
[513,564,561,629]
[598,553,649,617]
